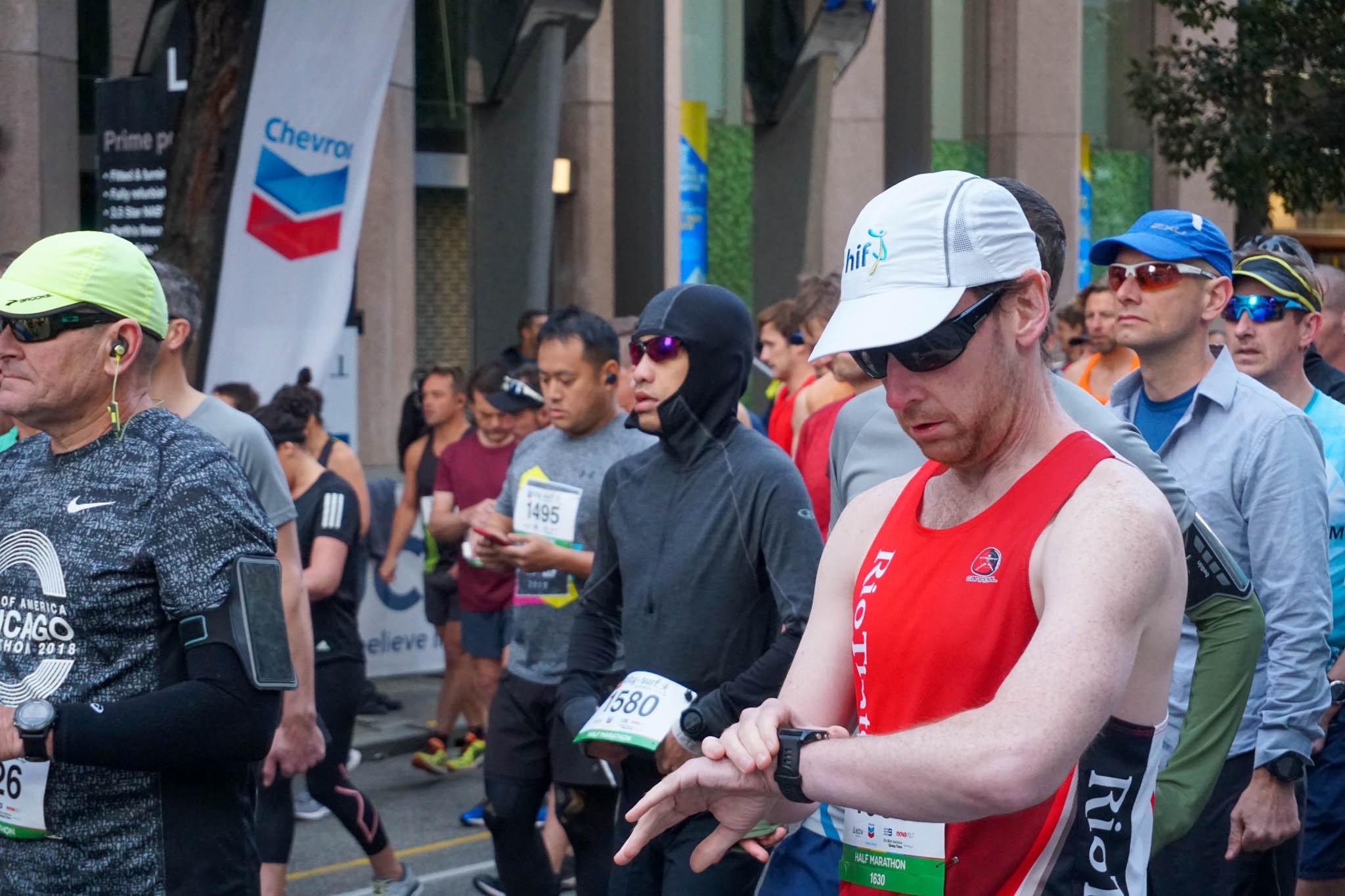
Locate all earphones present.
[110,335,129,357]
[605,373,617,385]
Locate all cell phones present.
[236,558,297,689]
[473,527,511,546]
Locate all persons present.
[375,171,1345,896]
[128,261,425,896]
[0,229,290,896]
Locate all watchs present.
[1264,754,1303,783]
[15,698,56,762]
[773,728,830,803]
[1328,677,1345,705]
[680,707,713,742]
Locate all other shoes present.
[460,804,486,827]
[370,862,424,896]
[411,736,448,777]
[473,871,506,896]
[444,730,485,770]
[357,691,402,715]
[293,796,331,820]
[346,747,361,772]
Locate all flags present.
[205,0,401,412]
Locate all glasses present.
[1108,263,1217,293]
[501,376,544,404]
[1235,234,1299,258]
[629,336,684,365]
[788,332,804,345]
[846,287,1009,380]
[0,313,119,343]
[1220,294,1308,325]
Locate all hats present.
[147,256,203,330]
[1088,208,1234,282]
[1230,255,1323,314]
[805,171,1042,363]
[0,230,169,341]
[1233,232,1315,274]
[1068,333,1089,347]
[486,385,544,414]
